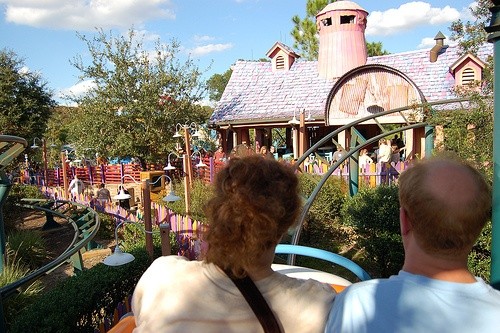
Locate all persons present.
[212,147,224,158]
[130,156,337,333]
[324,158,500,333]
[255,135,406,164]
[68,175,85,198]
[117,186,130,210]
[97,183,112,203]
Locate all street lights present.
[173,121,202,188]
[163,151,208,216]
[102,220,172,266]
[112,174,182,263]
[31,136,58,191]
